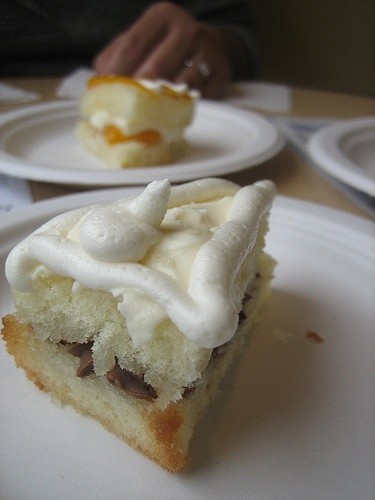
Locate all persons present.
[93,0,233,103]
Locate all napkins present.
[56,68,293,114]
[0,82,43,105]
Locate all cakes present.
[73,72,200,172]
[0,176,283,474]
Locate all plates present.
[0,187,375,499]
[0,99,293,187]
[304,111,375,198]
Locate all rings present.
[184,50,211,77]
